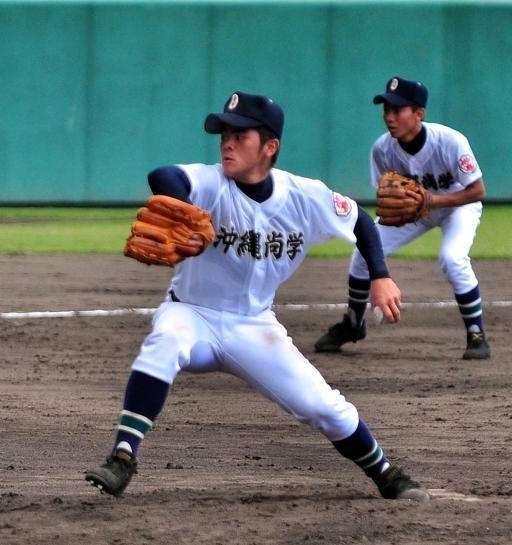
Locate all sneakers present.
[462,331,491,361]
[84,447,139,497]
[314,313,367,354]
[374,464,421,499]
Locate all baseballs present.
[374,306,389,324]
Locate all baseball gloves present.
[124,194,215,267]
[376,171,433,227]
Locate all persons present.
[315,76,492,359]
[86,91,432,500]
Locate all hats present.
[373,75,428,109]
[203,90,285,139]
